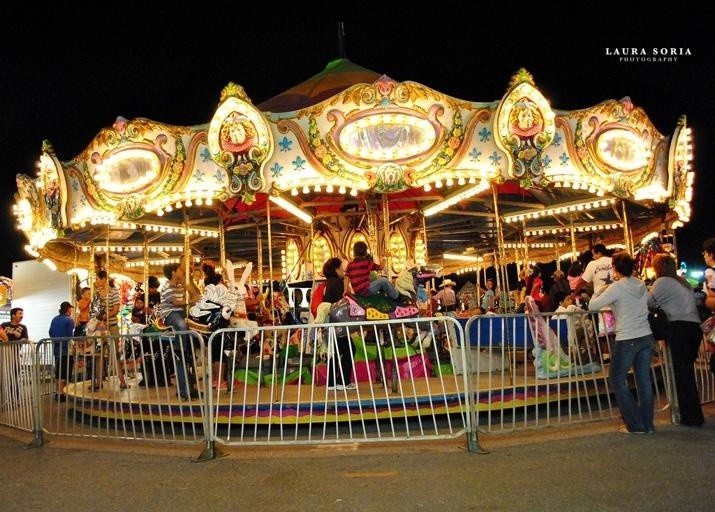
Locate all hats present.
[439,279,456,287]
[60,302,74,309]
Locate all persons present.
[67,236,715,402]
[586,249,661,439]
[0,307,28,409]
[48,300,75,403]
[0,325,8,343]
[645,252,706,429]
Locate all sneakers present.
[88,383,103,391]
[173,350,186,360]
[337,383,359,390]
[177,389,203,398]
[398,293,410,302]
[54,393,66,402]
[120,382,130,389]
[328,386,334,390]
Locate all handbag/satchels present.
[648,309,675,341]
[86,318,99,333]
